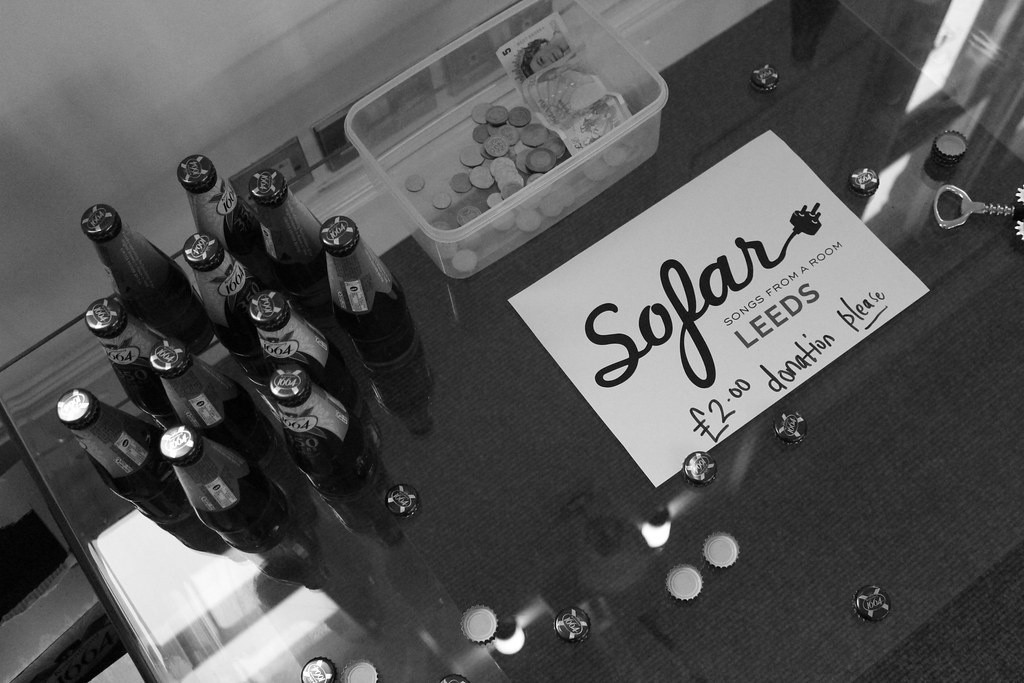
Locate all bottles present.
[56,154,418,554]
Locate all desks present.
[0,1,1024,683]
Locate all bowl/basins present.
[343,0,669,279]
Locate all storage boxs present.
[344,0,668,278]
